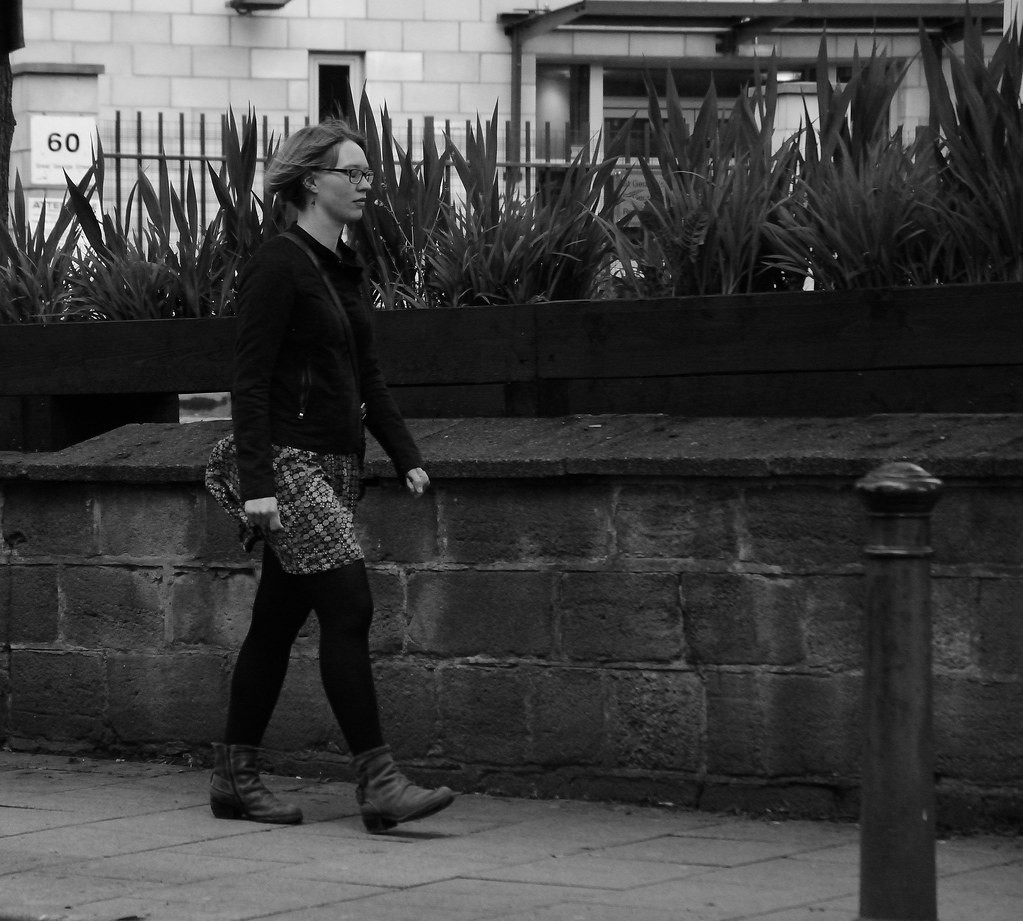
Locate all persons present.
[204,121,455,833]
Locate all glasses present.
[310,165,375,185]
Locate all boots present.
[353,747,452,835]
[209,741,302,825]
